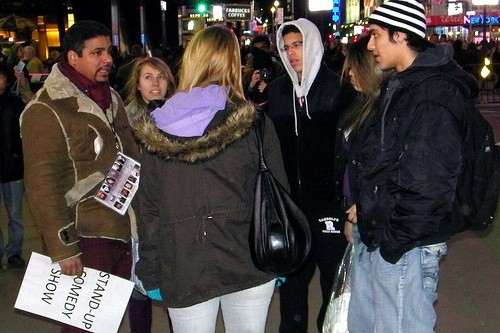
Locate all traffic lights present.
[198,4,205,14]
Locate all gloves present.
[148,289,162,301]
[276,277,285,286]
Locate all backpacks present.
[413,93,499,232]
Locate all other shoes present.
[8,255,25,267]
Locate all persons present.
[429,34,500,92]
[114,44,143,92]
[0,62,26,268]
[114,202,123,209]
[262,0,479,333]
[118,182,133,202]
[98,192,106,199]
[47,50,58,64]
[128,164,140,183]
[109,155,126,178]
[137,25,291,333]
[123,57,176,126]
[0,41,44,74]
[102,178,115,193]
[19,20,142,333]
[241,35,283,111]
[108,45,124,88]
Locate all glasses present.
[281,42,303,53]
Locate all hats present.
[368,0,426,37]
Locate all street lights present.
[273,0,279,31]
[271,6,275,34]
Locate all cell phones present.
[17,61,25,72]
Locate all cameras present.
[259,68,272,79]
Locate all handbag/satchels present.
[323,243,355,333]
[250,109,310,275]
